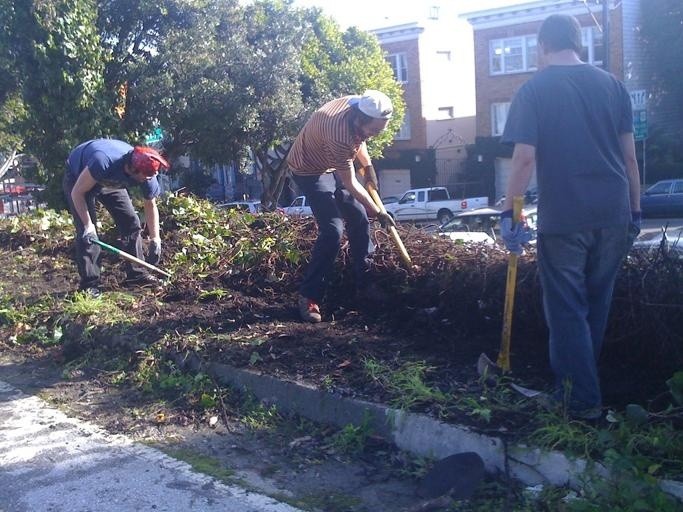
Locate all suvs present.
[426,222,499,250]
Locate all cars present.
[217,200,262,215]
[382,194,404,204]
[283,196,314,217]
[434,206,538,246]
[502,183,538,205]
[639,178,682,216]
[633,226,682,256]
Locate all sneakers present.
[126,274,163,288]
[79,285,108,297]
[298,295,321,323]
[348,261,371,278]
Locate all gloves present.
[148,238,161,265]
[378,212,395,232]
[500,209,532,253]
[631,212,642,233]
[82,225,97,247]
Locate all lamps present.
[477,152,483,163]
[415,152,421,162]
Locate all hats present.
[131,147,169,175]
[347,90,394,118]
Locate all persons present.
[205,179,224,202]
[282,88,396,322]
[62,139,172,298]
[498,12,643,421]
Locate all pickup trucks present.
[384,186,490,225]
